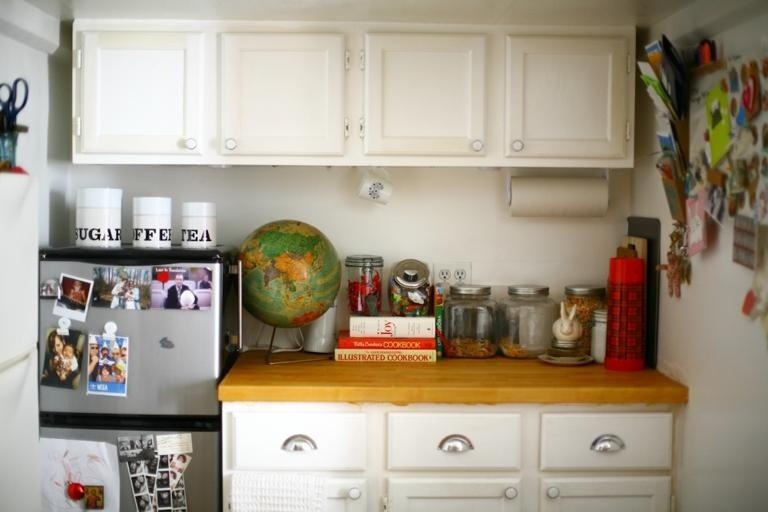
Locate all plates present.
[538,353,593,365]
[545,351,587,361]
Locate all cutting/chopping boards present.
[617,217,661,369]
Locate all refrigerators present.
[35,246,240,512]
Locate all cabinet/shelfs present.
[357,23,637,169]
[71,20,353,165]
[218,404,689,512]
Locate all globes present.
[232,220,342,364]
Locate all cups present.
[344,254,383,315]
[356,167,395,207]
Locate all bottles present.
[389,258,431,315]
[133,197,172,248]
[604,258,645,369]
[180,202,218,249]
[561,284,607,355]
[444,282,498,356]
[74,187,123,247]
[498,285,558,358]
[590,311,607,361]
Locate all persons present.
[200,275,212,289]
[89,340,127,383]
[129,455,186,512]
[163,274,199,309]
[111,278,140,309]
[70,281,87,303]
[42,331,79,388]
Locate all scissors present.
[0,78,28,131]
